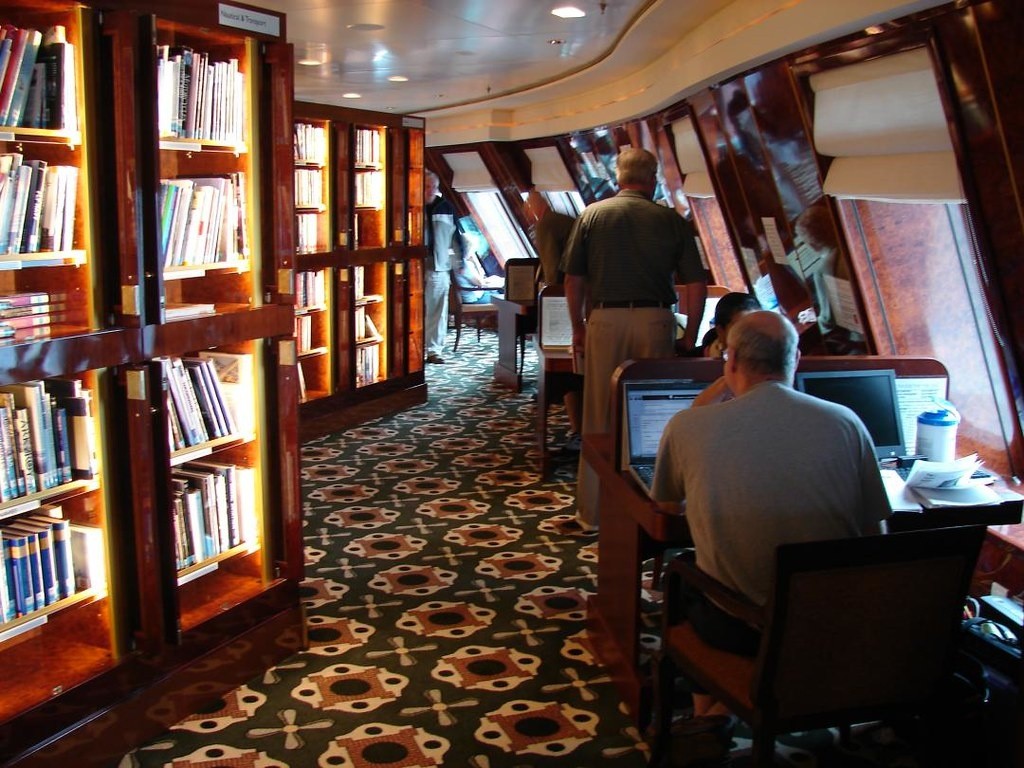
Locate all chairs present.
[647,523,989,768]
[448,269,506,352]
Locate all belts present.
[593,300,669,310]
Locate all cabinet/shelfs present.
[0,0,429,768]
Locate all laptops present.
[622,379,714,495]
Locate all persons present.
[717,73,868,356]
[424,169,467,364]
[572,127,668,207]
[681,292,763,357]
[649,313,893,719]
[450,233,505,304]
[553,148,708,537]
[528,190,581,438]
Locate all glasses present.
[722,348,737,362]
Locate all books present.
[156,44,256,571]
[0,25,103,626]
[291,121,381,404]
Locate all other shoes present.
[427,355,444,364]
[576,508,599,531]
[659,714,732,765]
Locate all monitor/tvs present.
[797,370,908,460]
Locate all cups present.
[917,394,961,461]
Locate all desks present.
[490,258,1024,736]
